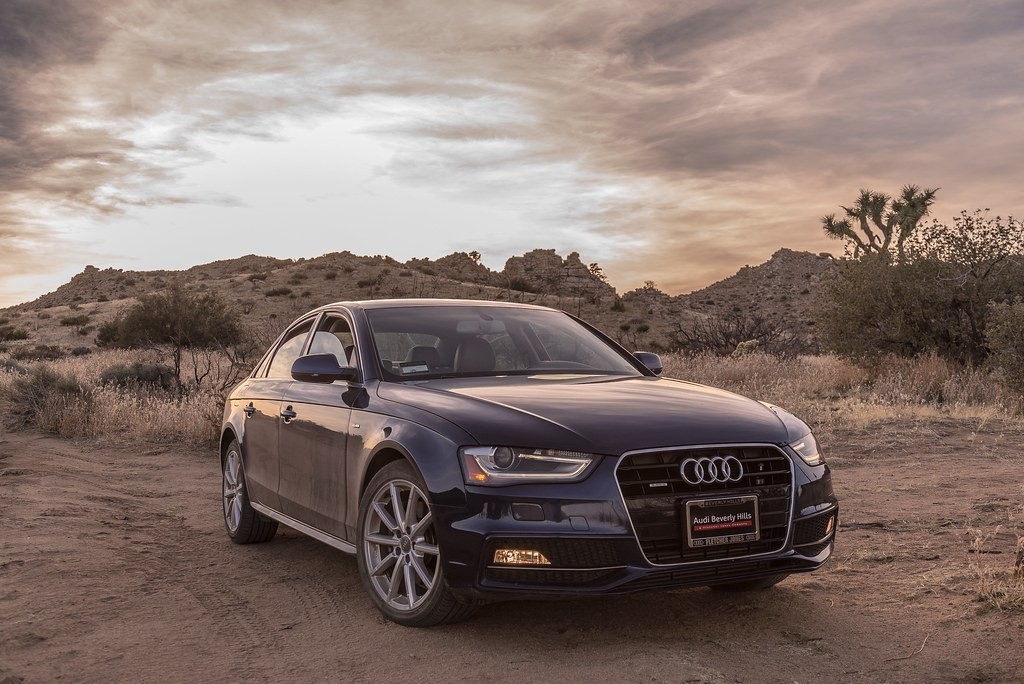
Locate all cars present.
[218,298,840,628]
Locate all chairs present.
[454,337,496,373]
[405,346,441,375]
